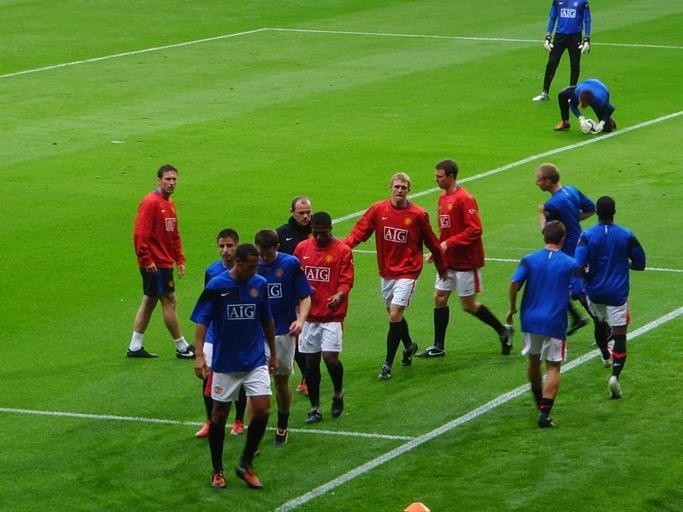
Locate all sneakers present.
[608,375,622,399]
[195,420,212,438]
[501,325,514,356]
[297,382,309,395]
[210,469,227,489]
[415,345,445,358]
[532,93,549,101]
[555,120,569,130]
[302,407,322,424]
[273,429,289,446]
[538,415,555,428]
[175,345,195,358]
[234,462,263,488]
[126,347,158,359]
[401,342,418,366]
[378,363,392,379]
[332,391,344,417]
[602,345,613,366]
[566,315,589,337]
[229,419,245,435]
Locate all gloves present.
[544,36,555,52]
[581,40,590,54]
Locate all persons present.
[292,211,355,424]
[255,228,314,447]
[274,197,313,396]
[189,243,277,489]
[194,227,246,438]
[532,1,592,105]
[573,195,646,400]
[552,78,617,135]
[341,171,450,381]
[506,219,588,428]
[534,162,614,344]
[126,164,195,358]
[414,159,514,358]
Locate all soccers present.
[584,119,597,134]
